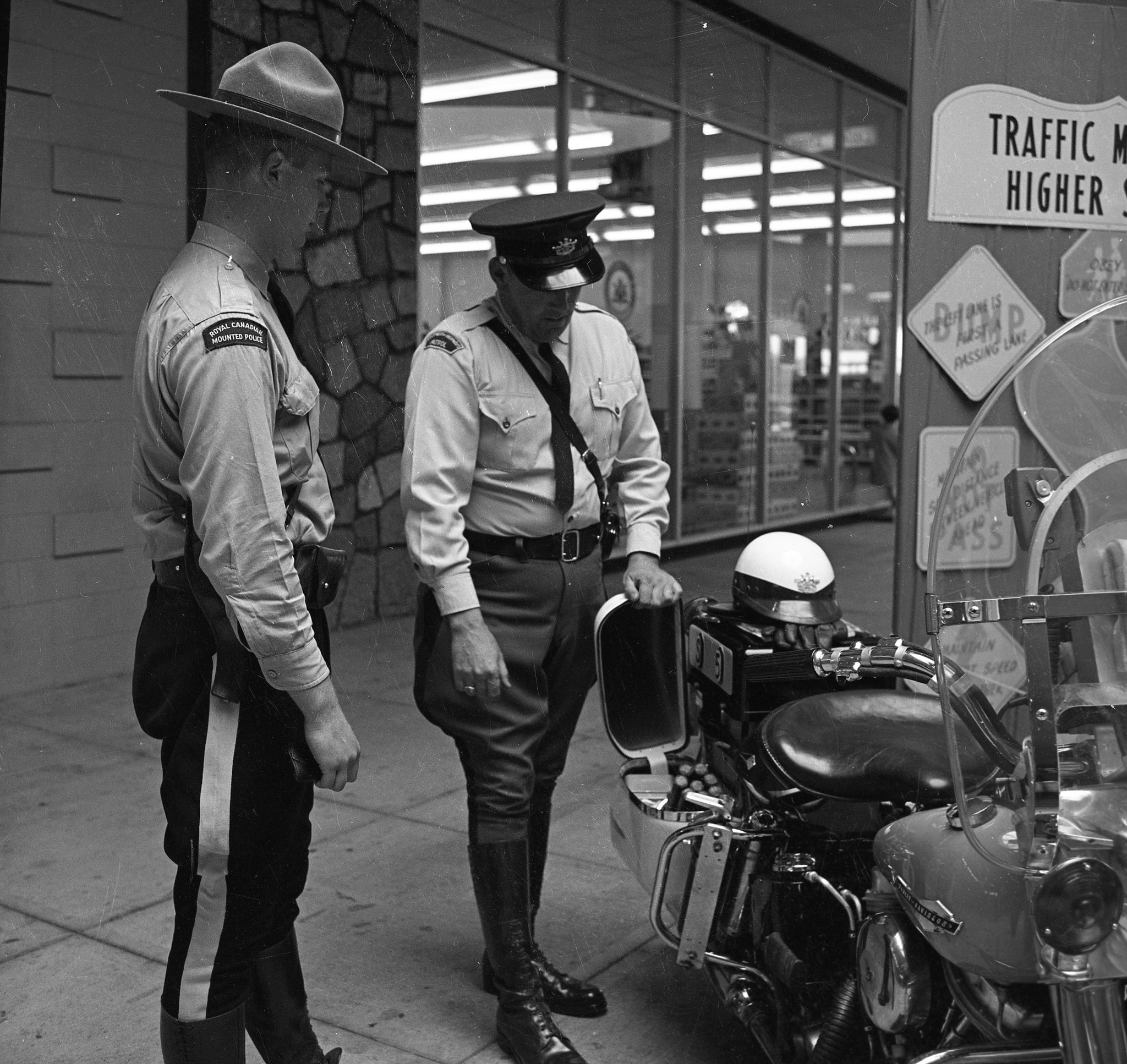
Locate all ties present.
[267,271,306,368]
[538,342,574,515]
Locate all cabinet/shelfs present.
[795,374,882,461]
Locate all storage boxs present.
[684,594,898,746]
[594,587,730,902]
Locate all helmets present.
[732,531,842,625]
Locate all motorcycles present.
[591,294,1127,1063]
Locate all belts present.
[154,557,188,591]
[463,523,603,562]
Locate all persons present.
[399,189,686,1064]
[879,405,900,507]
[125,38,394,1064]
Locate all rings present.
[463,686,476,696]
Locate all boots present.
[482,802,607,1017]
[469,839,587,1064]
[245,926,342,1064]
[160,993,246,1064]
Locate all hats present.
[155,42,388,175]
[468,191,605,291]
